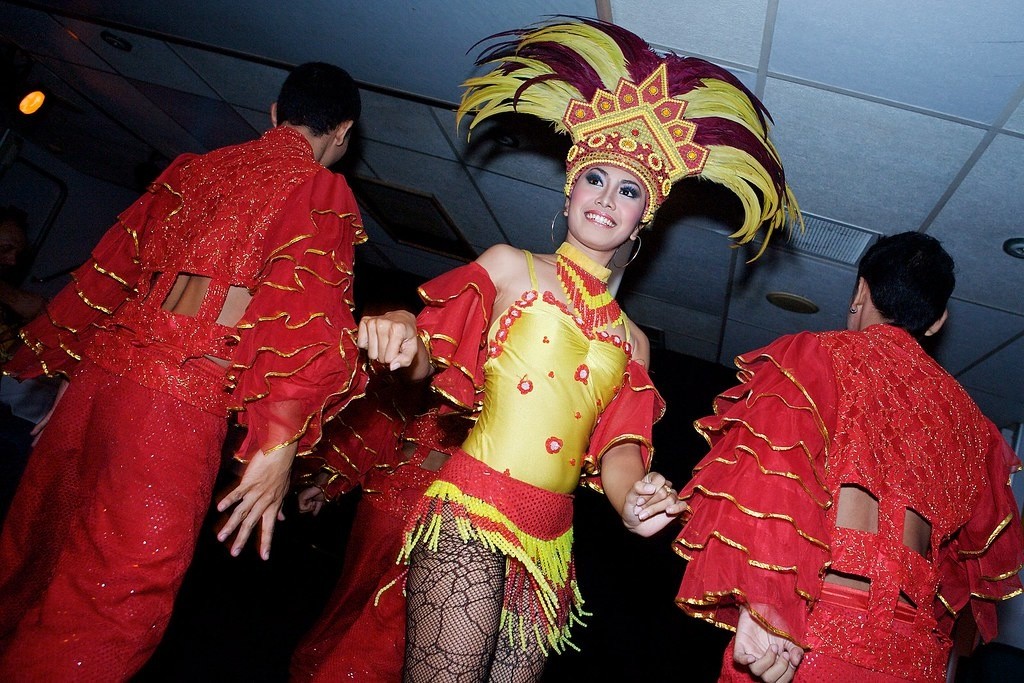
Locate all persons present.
[673,231,1024,683]
[0,12,806,683]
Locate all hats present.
[454,10,803,264]
